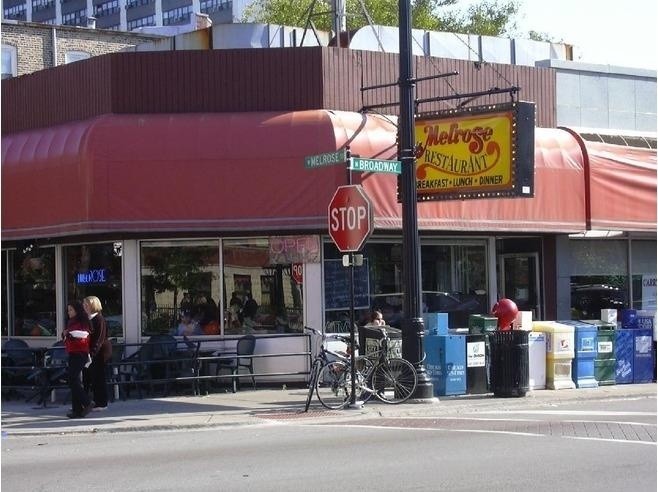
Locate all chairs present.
[1,334,256,406]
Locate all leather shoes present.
[91,403,108,411]
[66,411,86,419]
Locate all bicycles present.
[304,327,419,411]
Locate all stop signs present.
[328,184,374,252]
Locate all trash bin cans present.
[486,330,532,398]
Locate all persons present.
[182,290,258,314]
[82,296,112,412]
[179,310,202,334]
[14,314,41,335]
[62,301,97,419]
